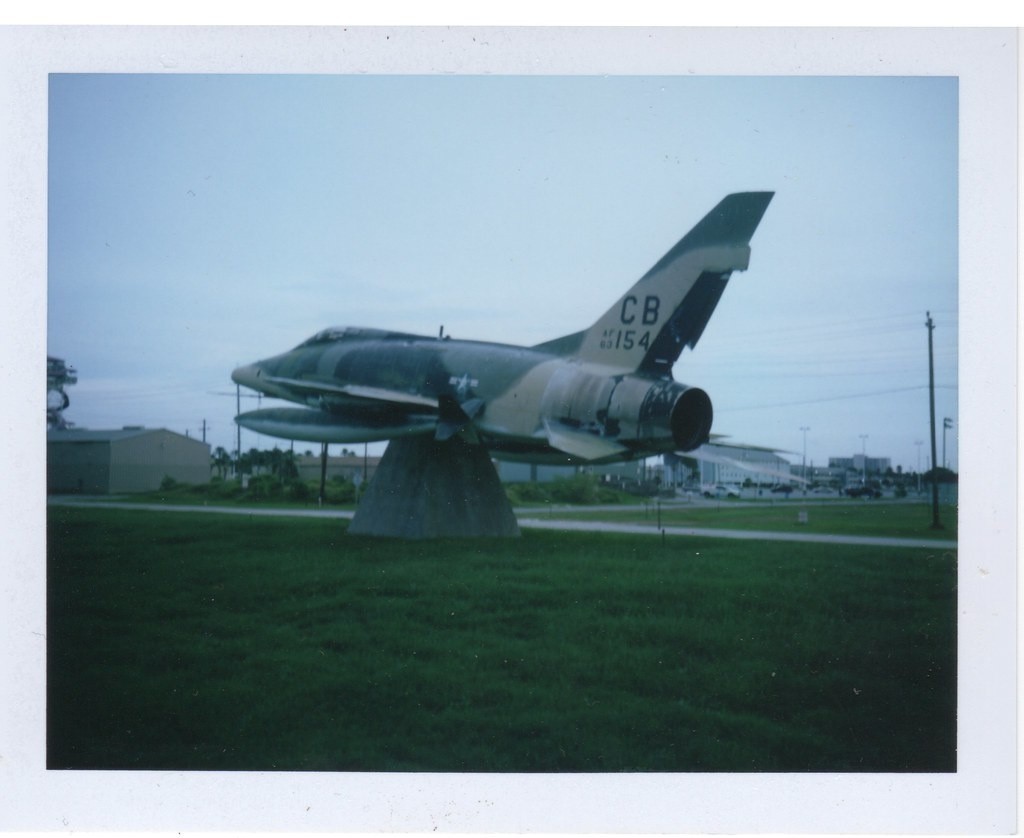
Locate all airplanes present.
[228,188,809,484]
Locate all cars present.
[699,483,742,501]
[770,482,795,494]
[841,483,882,500]
[814,485,835,494]
